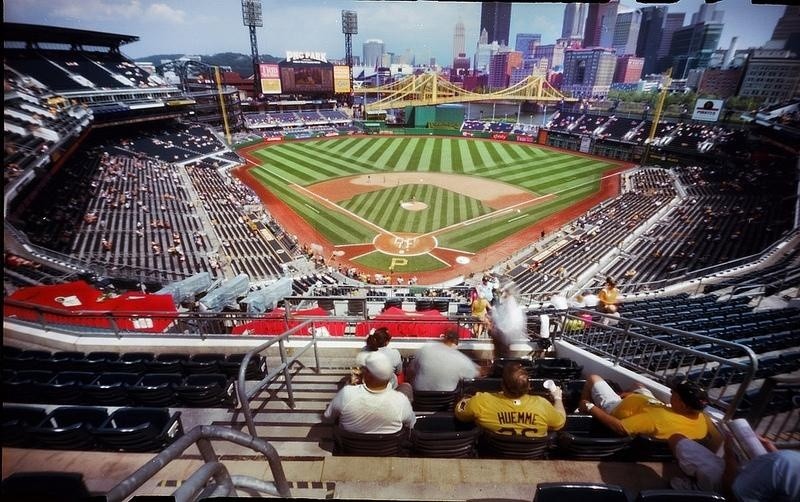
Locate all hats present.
[671,376,710,411]
[355,351,394,381]
[605,305,617,312]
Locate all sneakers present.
[670,477,700,491]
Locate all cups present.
[543,380,557,394]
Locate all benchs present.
[0,345,266,454]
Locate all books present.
[707,417,770,466]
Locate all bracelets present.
[587,402,595,415]
[551,396,564,401]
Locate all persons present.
[411,193,417,208]
[272,74,800,153]
[321,350,417,441]
[483,278,534,360]
[666,414,799,502]
[407,327,481,397]
[620,154,798,283]
[4,55,272,325]
[273,199,620,330]
[574,372,709,441]
[454,362,570,438]
[352,326,408,387]
